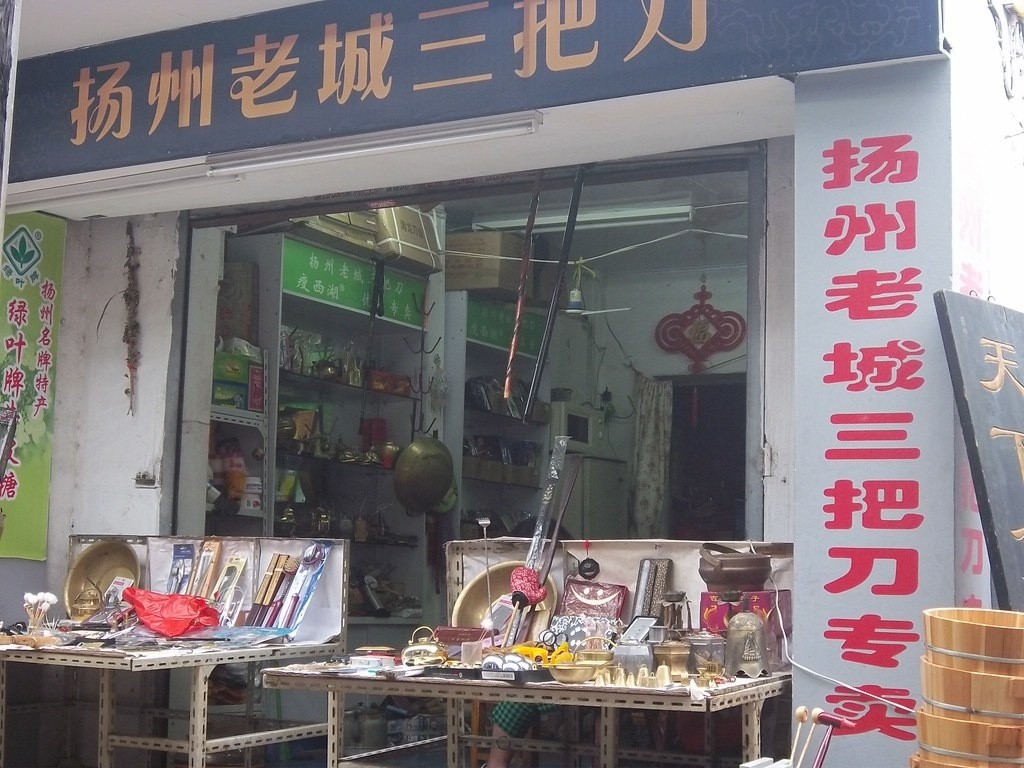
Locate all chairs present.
[470,701,563,768]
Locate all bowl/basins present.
[452,561,558,628]
[63,540,140,618]
[548,667,596,684]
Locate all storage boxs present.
[214,338,264,411]
[445,232,584,299]
[699,589,790,639]
[298,207,443,277]
[216,262,260,348]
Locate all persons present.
[477,514,576,767]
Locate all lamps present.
[468,204,699,234]
[204,111,546,180]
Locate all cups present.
[461,642,483,665]
[649,625,668,641]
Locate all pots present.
[394,431,453,517]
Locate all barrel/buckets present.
[916,708,1024,768]
[909,751,976,768]
[920,655,1024,725]
[923,608,1024,676]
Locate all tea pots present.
[401,626,449,667]
[572,637,617,681]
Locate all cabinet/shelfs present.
[168,291,442,768]
[459,340,553,539]
[259,538,793,768]
[0,536,349,768]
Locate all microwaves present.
[550,401,608,457]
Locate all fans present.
[505,265,632,326]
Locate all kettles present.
[344,702,386,748]
